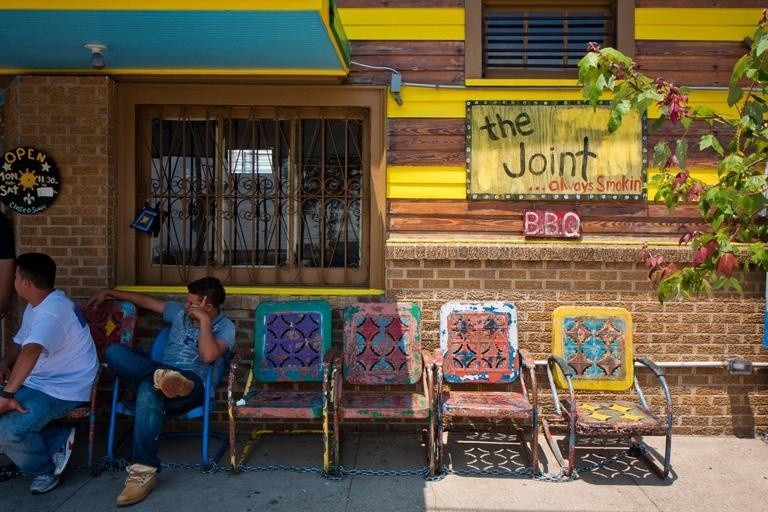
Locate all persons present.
[0,208,14,320]
[76,275,238,508]
[0,252,101,495]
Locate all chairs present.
[330,302,434,479]
[224,300,332,479]
[539,305,673,482]
[54,295,138,479]
[433,300,538,477]
[105,327,228,476]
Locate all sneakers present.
[49,427,76,476]
[30,474,61,495]
[117,464,158,506]
[155,369,195,399]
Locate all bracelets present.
[0,390,16,400]
